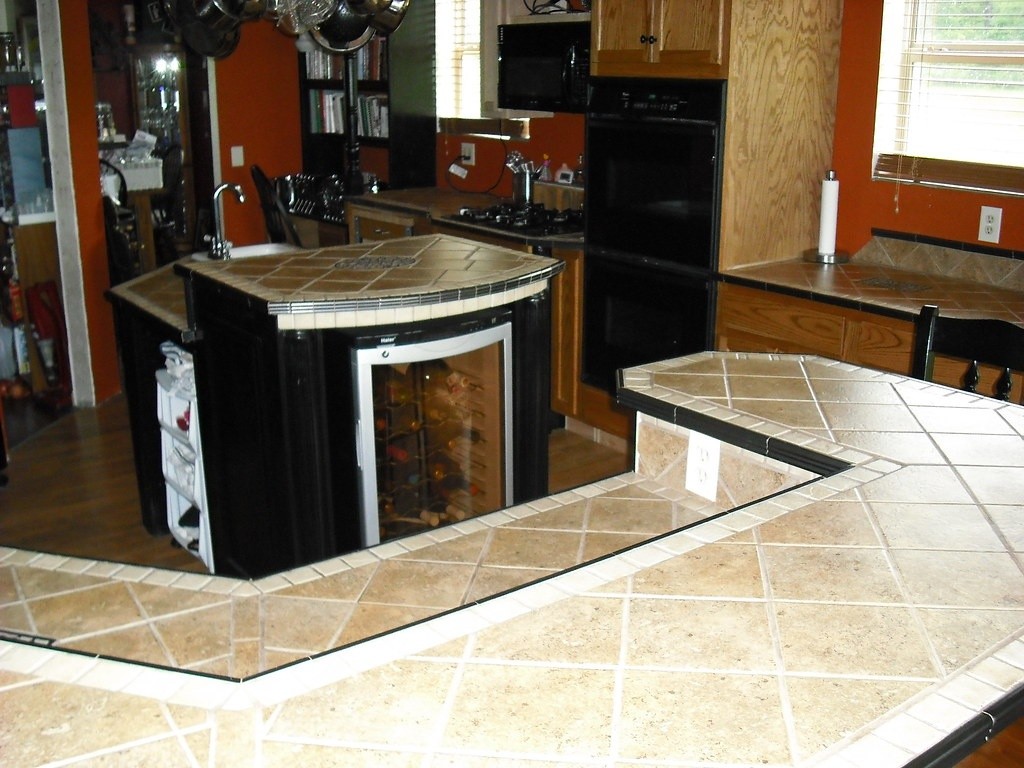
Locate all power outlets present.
[461,142,476,167]
[977,206,1003,244]
[231,146,244,166]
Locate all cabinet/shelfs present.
[592,0,731,81]
[299,0,438,188]
[172,232,566,578]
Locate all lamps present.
[145,0,412,61]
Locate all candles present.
[819,177,839,256]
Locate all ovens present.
[580,75,727,393]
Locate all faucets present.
[201,181,246,263]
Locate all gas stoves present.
[430,202,583,237]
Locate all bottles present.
[574,156,585,188]
[372,360,480,534]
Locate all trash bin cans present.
[155,221,178,262]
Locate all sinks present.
[191,243,304,261]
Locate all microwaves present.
[497,21,590,113]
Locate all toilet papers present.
[818,178,840,256]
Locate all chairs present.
[250,163,305,250]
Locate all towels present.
[159,340,196,397]
[168,441,194,499]
[177,505,199,551]
[177,406,190,438]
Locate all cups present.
[511,171,530,203]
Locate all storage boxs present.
[150,367,206,565]
[99,158,164,197]
